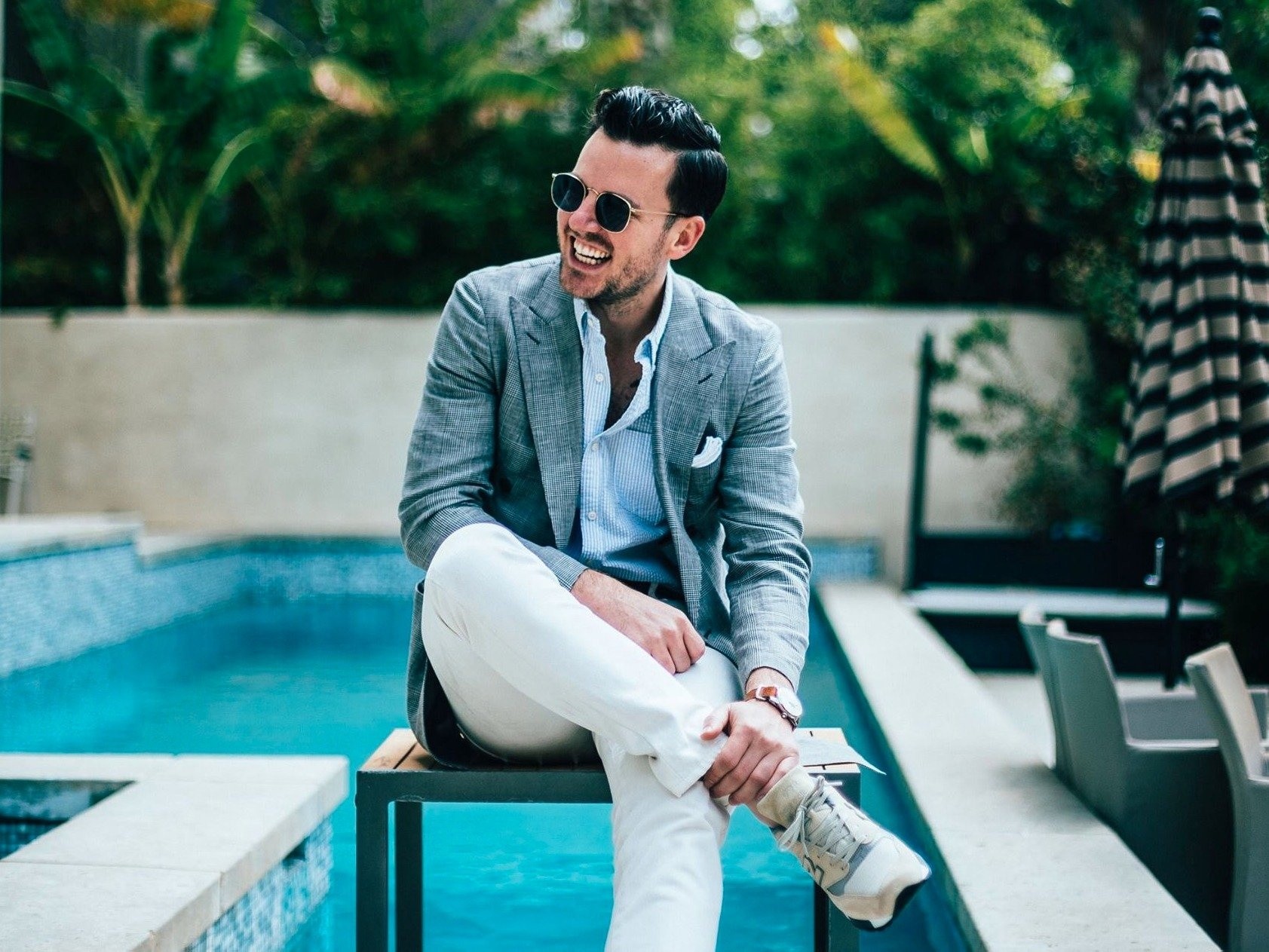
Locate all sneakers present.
[755,762,932,932]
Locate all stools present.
[356,729,862,951]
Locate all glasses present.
[550,173,686,234]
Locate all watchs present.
[742,684,803,733]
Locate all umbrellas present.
[1114,25,1268,691]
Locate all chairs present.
[1019,604,1269,952]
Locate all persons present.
[394,82,934,951]
